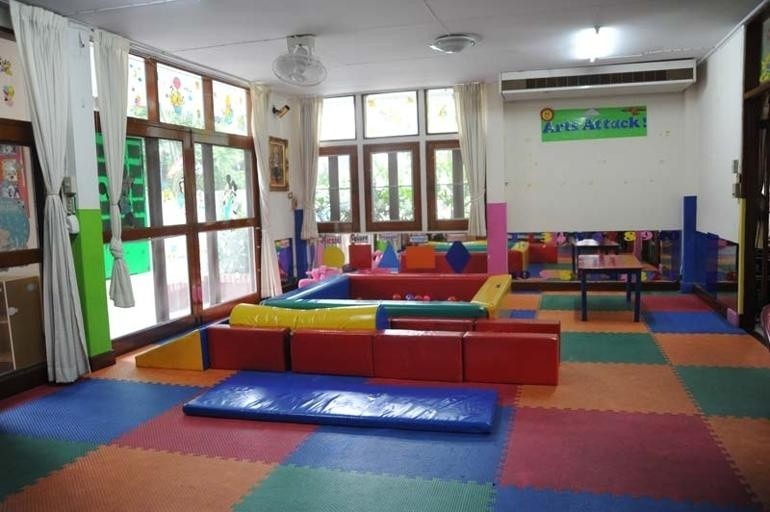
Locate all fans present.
[272,33,327,86]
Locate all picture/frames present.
[268,136,289,191]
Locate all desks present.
[571,237,644,322]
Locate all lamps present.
[427,35,476,54]
